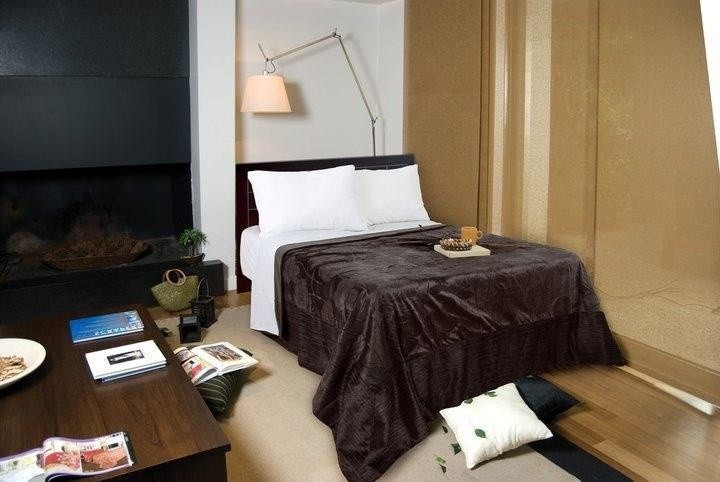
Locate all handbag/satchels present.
[151,270,201,312]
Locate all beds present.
[238,154,629,482]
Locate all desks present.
[1,304,231,481]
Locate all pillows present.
[247,164,366,232]
[440,386,553,470]
[354,163,431,224]
[511,372,580,423]
[188,346,251,416]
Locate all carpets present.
[152,303,632,482]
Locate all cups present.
[461,227,482,246]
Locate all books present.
[85,339,167,383]
[69,309,145,343]
[0,430,138,482]
[172,341,259,387]
[434,244,491,258]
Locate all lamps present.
[240,27,379,158]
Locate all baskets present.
[191,279,219,329]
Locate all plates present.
[0,337,46,388]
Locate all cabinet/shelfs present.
[0,0,203,326]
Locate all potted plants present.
[179,226,207,266]
